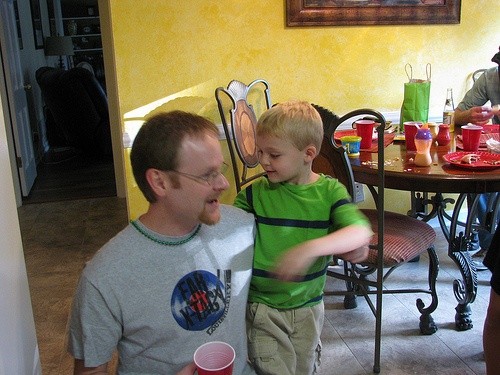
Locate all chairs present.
[215,79,273,193]
[310,104,440,374]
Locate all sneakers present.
[471,249,490,270]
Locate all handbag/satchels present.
[400,62,432,133]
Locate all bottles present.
[443,88,455,132]
[414,128,432,166]
[428,125,436,143]
[437,125,450,146]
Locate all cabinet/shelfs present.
[48,1,103,75]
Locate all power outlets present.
[353,183,365,202]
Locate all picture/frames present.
[285,0,462,27]
[29,0,45,49]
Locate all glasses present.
[165,162,229,185]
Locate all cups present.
[356,120,374,149]
[194,340,236,375]
[461,126,483,152]
[403,122,423,150]
[341,136,362,156]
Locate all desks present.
[336,128,500,335]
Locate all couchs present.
[36,65,113,161]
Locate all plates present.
[334,130,355,139]
[443,152,500,169]
[479,125,499,133]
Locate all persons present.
[233,100,371,375]
[66,110,369,375]
[455,44,500,271]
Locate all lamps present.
[45,33,74,70]
